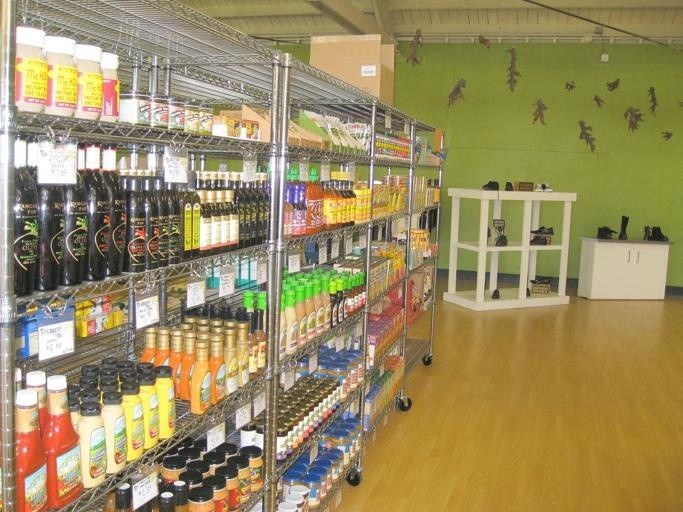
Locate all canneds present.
[119,89,260,142]
[159,375,340,512]
[374,174,426,218]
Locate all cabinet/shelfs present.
[152,44,375,511]
[443,188,674,308]
[346,99,444,378]
[200,47,414,446]
[3,2,278,511]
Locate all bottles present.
[14,133,370,511]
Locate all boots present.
[618,215,629,240]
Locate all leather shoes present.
[492,290,499,299]
[530,237,546,245]
[644,226,668,241]
[505,182,513,191]
[483,181,499,190]
[531,226,553,235]
[496,235,507,246]
[530,279,550,284]
